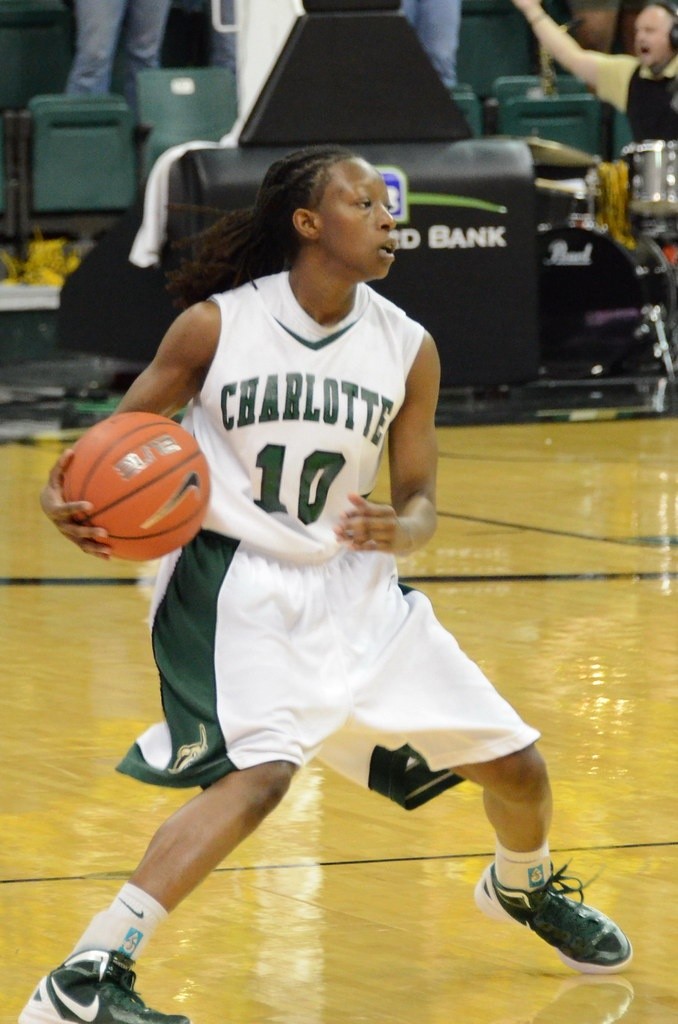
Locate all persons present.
[15,147,633,1023]
[398,1,462,90]
[508,0,678,143]
[62,0,170,136]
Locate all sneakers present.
[472,861,634,976]
[18,950,188,1024]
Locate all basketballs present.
[63,412,211,563]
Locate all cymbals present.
[487,134,596,166]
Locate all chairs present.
[1,1,632,264]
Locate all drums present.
[534,225,674,377]
[535,178,589,232]
[620,139,678,215]
